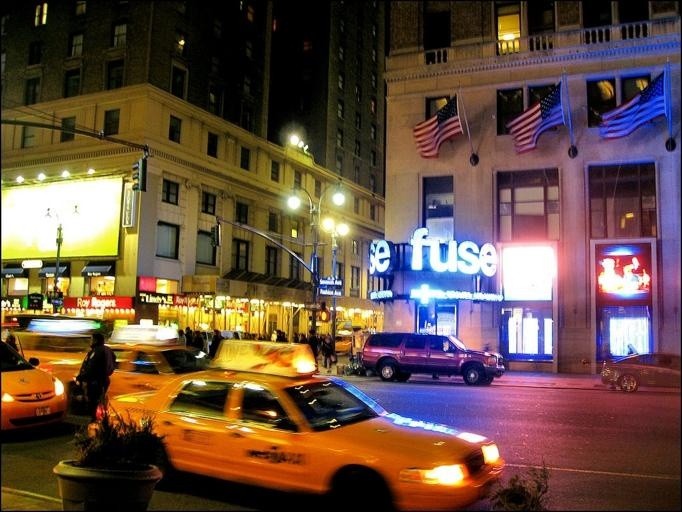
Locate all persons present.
[4,335,19,361]
[75,334,116,414]
[181,325,336,371]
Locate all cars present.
[334,335,351,354]
[1,317,92,385]
[39,324,220,411]
[0,339,68,432]
[598,350,682,393]
[99,335,508,511]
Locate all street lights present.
[319,216,351,362]
[286,176,346,366]
[52,221,65,314]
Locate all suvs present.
[361,331,510,386]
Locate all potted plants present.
[49,405,173,512]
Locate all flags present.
[599,68,666,139]
[505,80,567,153]
[413,93,464,159]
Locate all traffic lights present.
[204,308,210,313]
[131,157,148,192]
[210,223,222,247]
[319,308,330,323]
